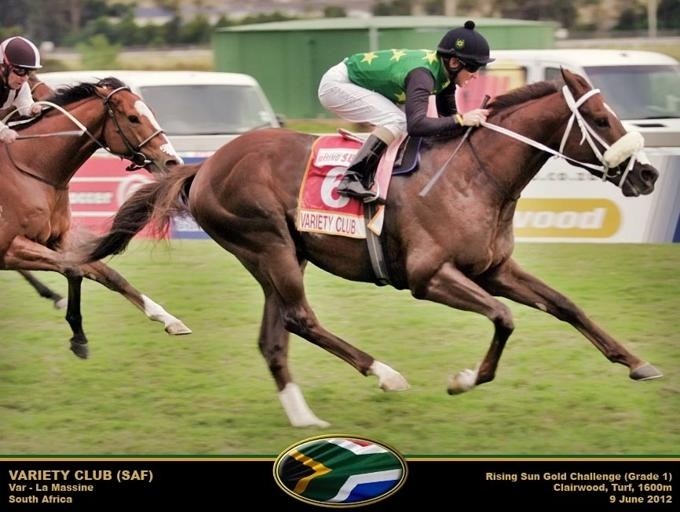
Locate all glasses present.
[12,67,33,76]
[463,61,481,72]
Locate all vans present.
[38,70,286,155]
[426,47,679,148]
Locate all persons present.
[0,35,44,142]
[316,20,498,206]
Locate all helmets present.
[435,27,496,64]
[0,35,43,70]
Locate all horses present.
[53,63,665,430]
[0,75,194,361]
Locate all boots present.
[337,134,388,204]
